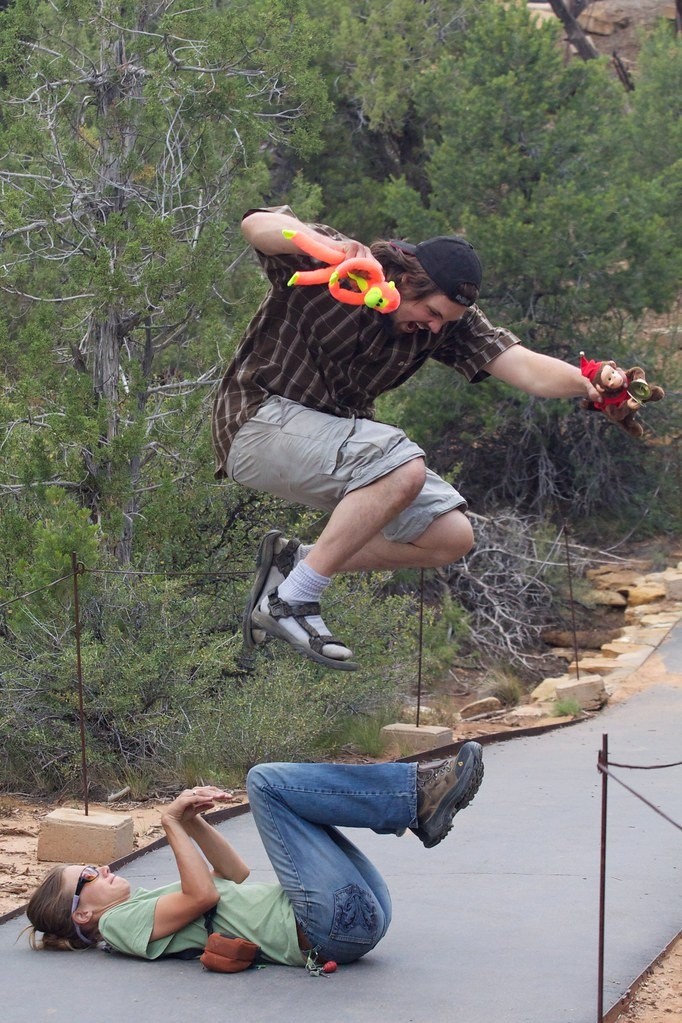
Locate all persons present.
[209,202,630,672]
[26,740,485,967]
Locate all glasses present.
[70,866,99,942]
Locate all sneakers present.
[408,741,484,848]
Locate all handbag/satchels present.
[200,932,261,974]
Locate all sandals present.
[249,586,359,670]
[243,530,302,650]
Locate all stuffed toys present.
[578,349,665,439]
[281,228,401,314]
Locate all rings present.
[191,788,198,797]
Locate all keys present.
[305,949,338,979]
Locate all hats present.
[390,235,481,307]
